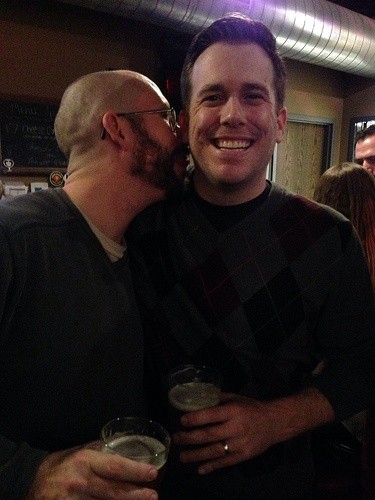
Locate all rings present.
[220,440,230,457]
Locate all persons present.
[354,124,375,179]
[124,12,375,500]
[312,162,375,286]
[0,69,189,500]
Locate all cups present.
[167,363,225,471]
[100,416,171,500]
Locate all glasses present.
[100,106,181,140]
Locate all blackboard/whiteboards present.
[0,99,69,168]
